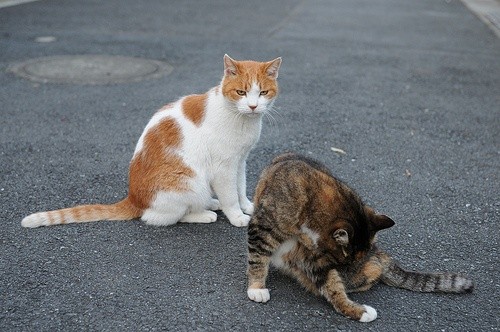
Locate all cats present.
[21,52,282,228]
[245,152,475,322]
